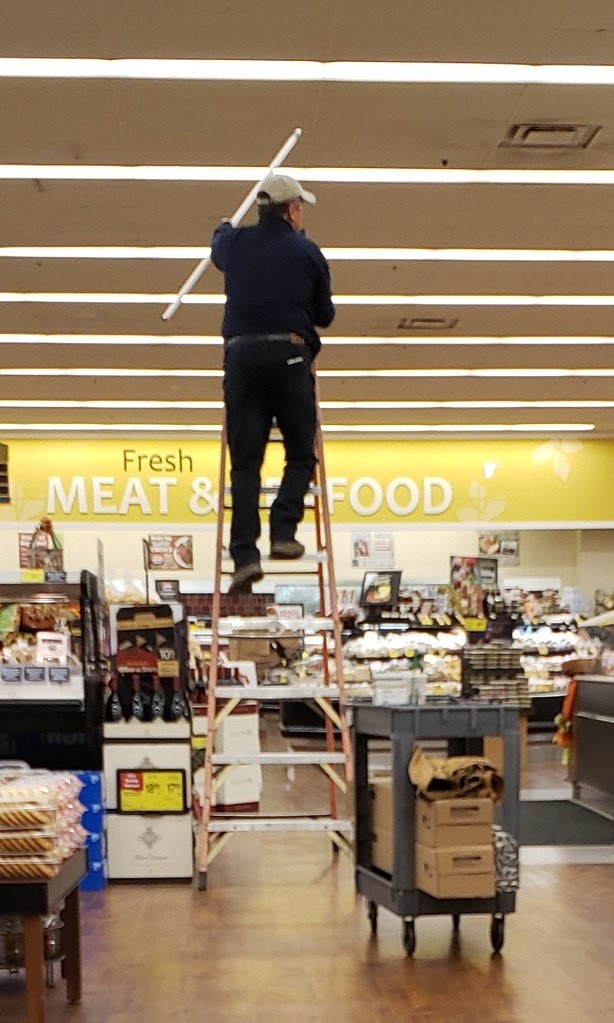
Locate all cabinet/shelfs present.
[0,569,614,891]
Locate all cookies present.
[0,785,58,879]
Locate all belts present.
[225,332,304,345]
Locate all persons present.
[211,175,337,590]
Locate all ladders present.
[196,360,354,891]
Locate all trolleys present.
[349,698,523,955]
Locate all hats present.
[256,176,316,205]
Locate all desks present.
[0,847,89,1023]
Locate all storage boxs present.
[416,798,497,846]
[371,829,395,876]
[410,848,496,898]
[367,780,394,829]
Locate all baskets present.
[27,529,64,570]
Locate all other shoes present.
[269,538,305,558]
[227,561,264,596]
